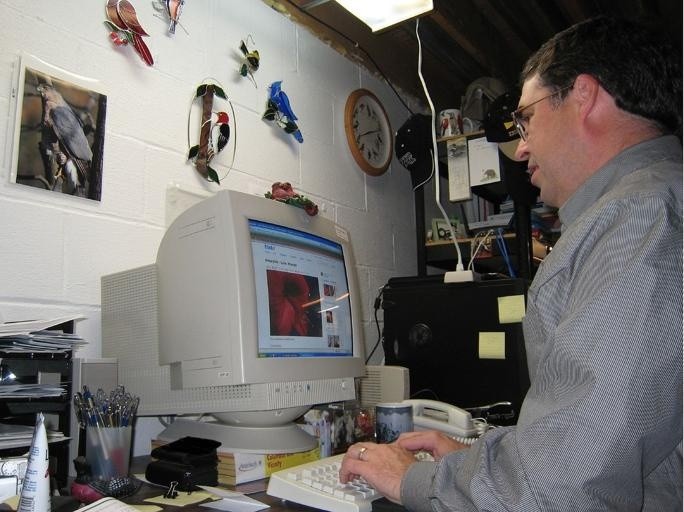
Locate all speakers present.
[362,364,411,405]
[69,359,118,477]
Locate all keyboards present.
[265,443,434,512]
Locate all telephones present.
[402,399,489,437]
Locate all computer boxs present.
[382,275,533,428]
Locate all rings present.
[358,447,367,460]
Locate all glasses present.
[510,84,573,142]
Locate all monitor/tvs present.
[99,187,368,457]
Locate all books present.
[469,196,562,232]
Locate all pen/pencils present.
[74,385,140,480]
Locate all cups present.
[437,109,462,138]
[84,428,131,480]
[374,401,414,443]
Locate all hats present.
[486,89,528,161]
[394,113,434,190]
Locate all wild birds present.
[36,83,95,196]
[440,112,459,136]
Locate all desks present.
[51,454,325,512]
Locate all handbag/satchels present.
[461,77,508,120]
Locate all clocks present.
[344,89,393,176]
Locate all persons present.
[446,222,458,240]
[337,14,683,512]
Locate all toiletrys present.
[17,413,55,512]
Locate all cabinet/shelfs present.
[0,320,74,499]
[411,129,561,280]
